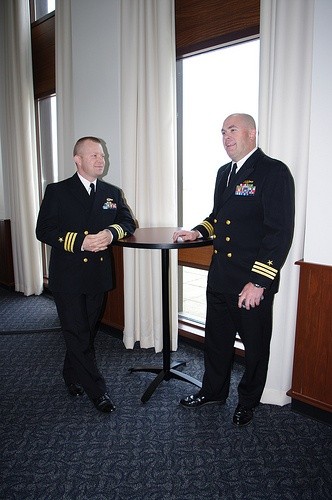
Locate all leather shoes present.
[178,391,226,408]
[94,392,116,413]
[232,402,254,426]
[67,383,86,397]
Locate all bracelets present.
[254,283,260,287]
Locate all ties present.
[228,163,237,185]
[90,183,96,207]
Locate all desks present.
[116,226,221,403]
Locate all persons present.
[172,113,296,426]
[37,137,136,411]
